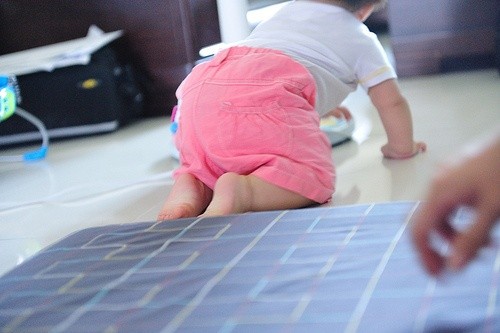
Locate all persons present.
[409,138,499,280]
[158,0,426,222]
